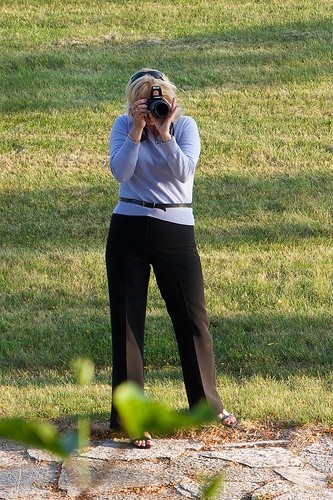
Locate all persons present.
[106,68,238,449]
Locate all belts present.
[121,197,192,212]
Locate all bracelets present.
[127,130,142,144]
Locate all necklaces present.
[146,125,161,145]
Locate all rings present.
[135,108,140,113]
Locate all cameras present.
[139,86,169,119]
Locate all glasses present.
[131,71,164,84]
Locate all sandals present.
[132,436,152,447]
[217,413,234,427]
